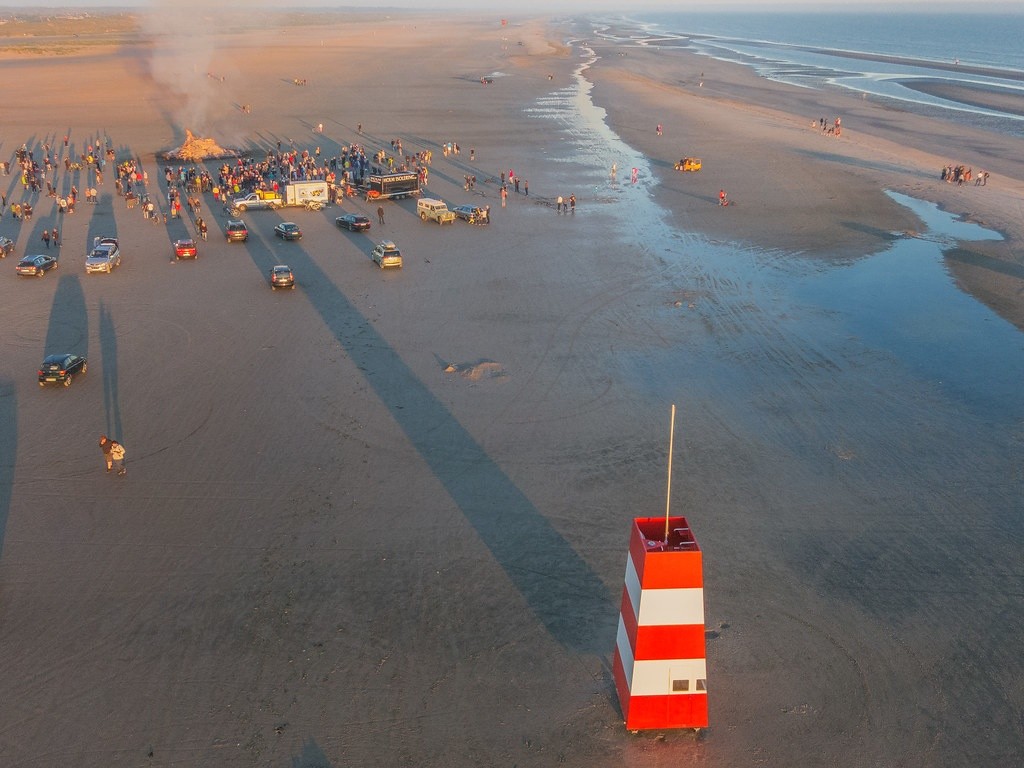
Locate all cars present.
[674,157,701,172]
[274,222,303,240]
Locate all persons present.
[941,165,990,186]
[0,136,159,224]
[52,228,62,247]
[811,117,843,138]
[162,74,577,241]
[610,80,731,207]
[98,436,126,475]
[41,231,50,249]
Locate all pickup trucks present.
[232,193,283,212]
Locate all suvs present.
[37,353,88,386]
[224,220,248,242]
[417,198,456,225]
[85,236,121,273]
[269,265,295,290]
[453,204,484,222]
[16,254,58,278]
[336,214,370,231]
[173,239,198,260]
[0,235,15,258]
[371,240,402,269]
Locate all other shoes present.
[117,468,127,475]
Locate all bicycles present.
[305,201,321,211]
